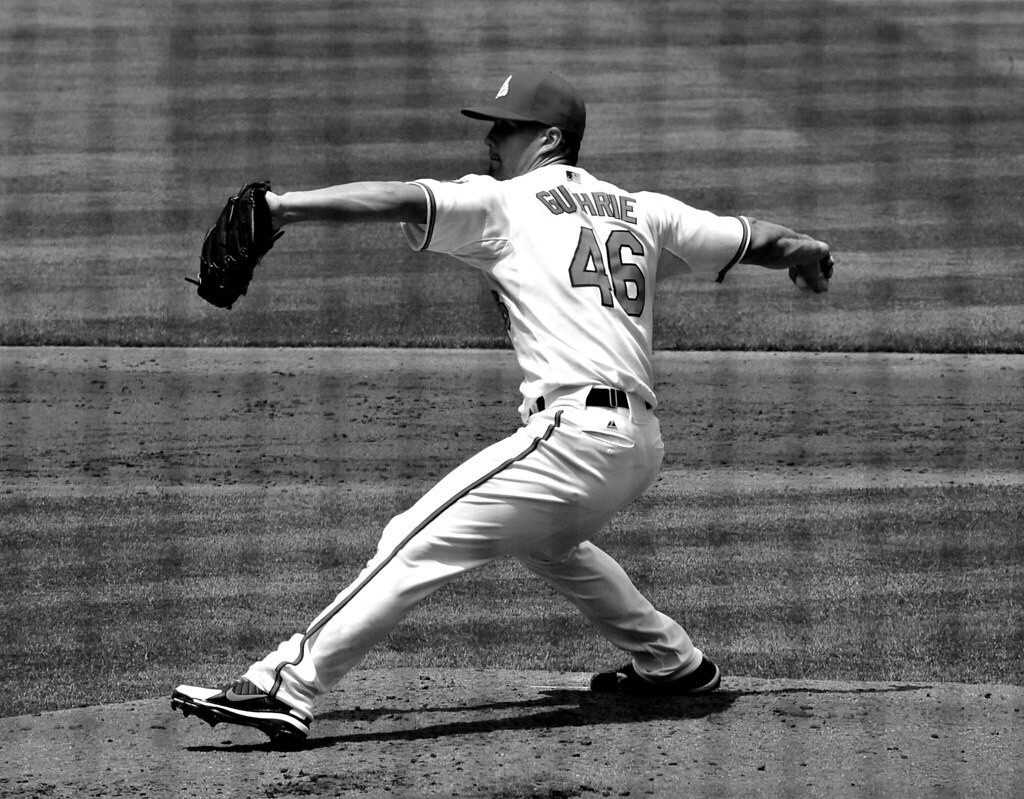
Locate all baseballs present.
[795,270,825,293]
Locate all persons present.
[170,71,836,744]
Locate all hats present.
[460,71,586,138]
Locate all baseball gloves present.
[181,179,287,312]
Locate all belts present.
[537,388,651,412]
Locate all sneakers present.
[171,678,311,749]
[592,648,721,698]
